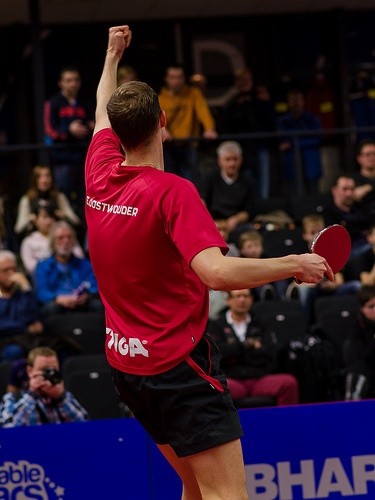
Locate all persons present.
[90,47,374,195]
[206,139,375,407]
[0,163,135,429]
[197,141,260,240]
[42,69,96,140]
[85,24,337,500]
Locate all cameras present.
[42,369,61,385]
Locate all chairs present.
[0,84,374,419]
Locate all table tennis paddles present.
[295,224,350,285]
